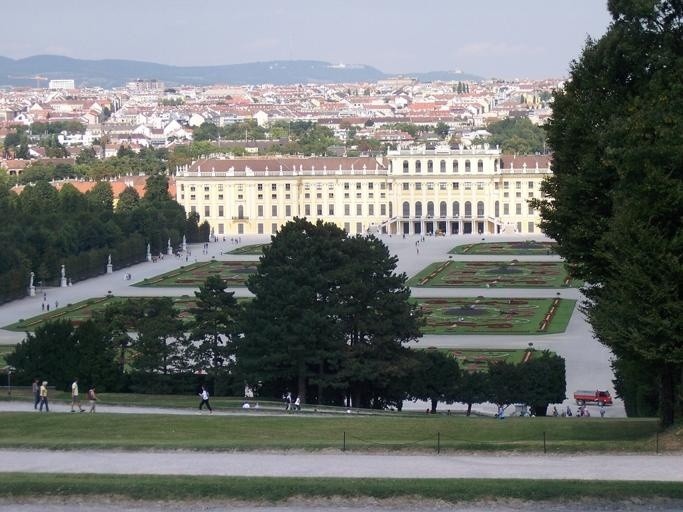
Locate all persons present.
[70,377,85,412]
[294,394,302,410]
[158,235,241,263]
[599,406,605,418]
[285,391,292,410]
[40,292,59,311]
[194,384,213,416]
[88,384,100,413]
[31,377,40,410]
[552,404,591,417]
[38,380,48,411]
[496,406,504,419]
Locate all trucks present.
[573,387,613,407]
